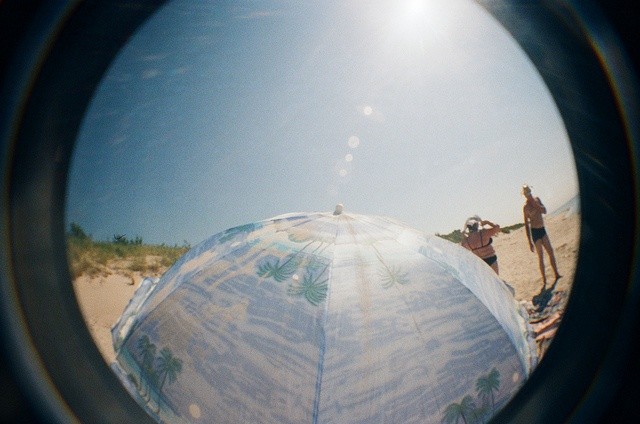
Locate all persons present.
[461,215,500,276]
[522,184,563,295]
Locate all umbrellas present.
[108,202,543,423]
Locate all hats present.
[519,184,534,196]
